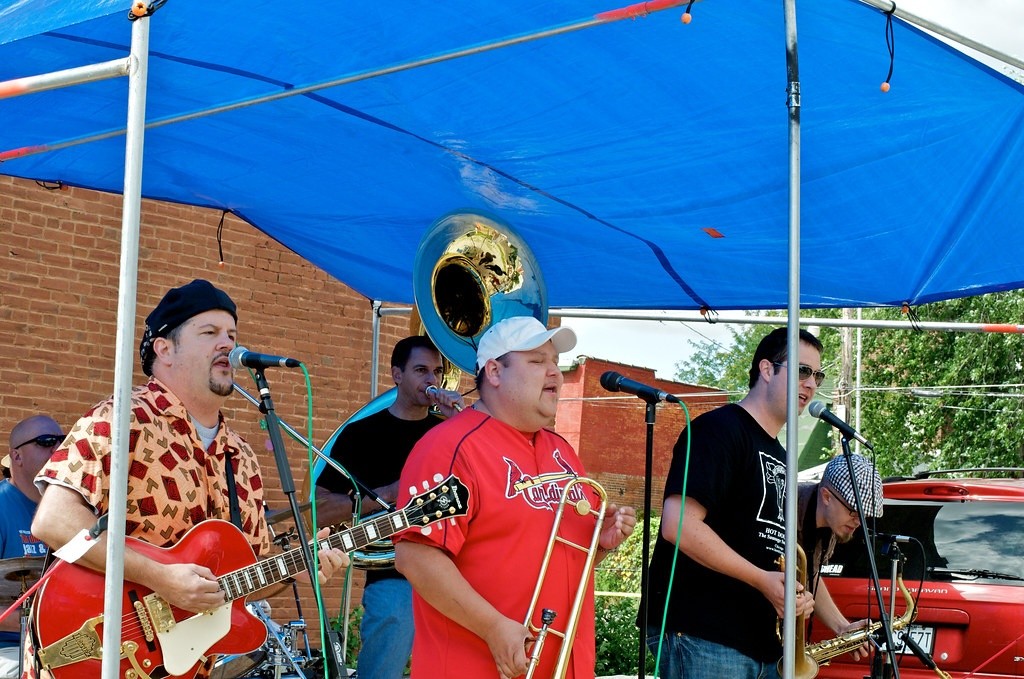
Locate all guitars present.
[28,472,473,679]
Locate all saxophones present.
[804,563,919,664]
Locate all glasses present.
[14,433,67,448]
[772,361,825,388]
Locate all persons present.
[390,317,637,679]
[311,336,466,679]
[636,328,823,679]
[0,415,68,650]
[797,453,883,661]
[30,281,351,679]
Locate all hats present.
[138,278,238,377]
[821,453,884,518]
[474,316,578,378]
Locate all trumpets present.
[779,539,820,679]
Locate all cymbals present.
[0,557,52,601]
[264,497,328,523]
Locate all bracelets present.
[597,545,607,553]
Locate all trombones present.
[504,476,608,679]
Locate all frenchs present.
[341,211,549,571]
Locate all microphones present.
[600,372,682,404]
[424,384,463,412]
[862,528,916,545]
[228,346,302,370]
[808,401,873,450]
